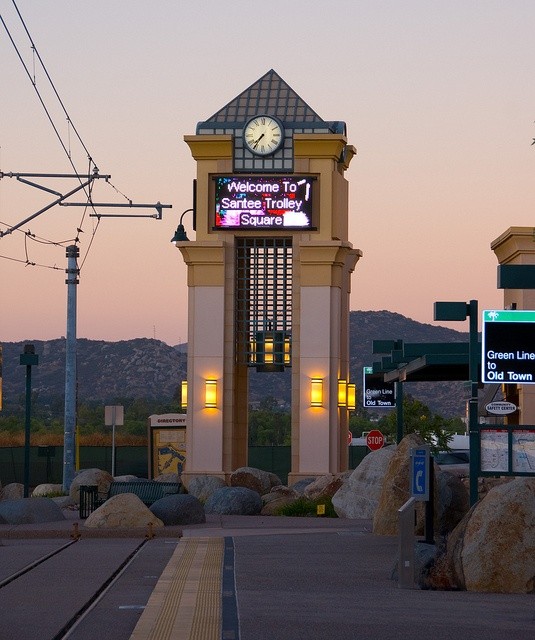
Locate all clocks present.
[243,113,284,156]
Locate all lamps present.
[346,382,355,410]
[249,337,257,364]
[204,378,217,407]
[338,379,347,407]
[311,377,323,407]
[283,338,291,364]
[181,380,187,408]
[264,337,274,364]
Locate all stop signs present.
[365,429,384,452]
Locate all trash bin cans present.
[79,485,98,519]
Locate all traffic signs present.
[363,366,396,408]
[410,445,429,501]
[481,310,535,384]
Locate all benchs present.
[94,482,182,510]
